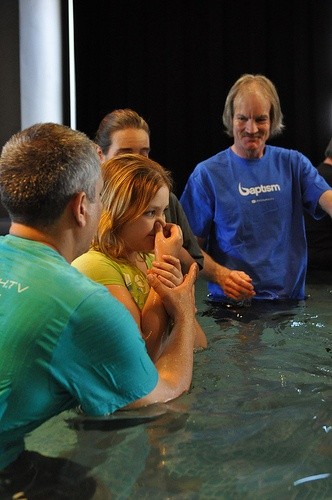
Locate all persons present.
[0,123,196,468]
[304,138,332,272]
[179,75,332,317]
[69,155,206,363]
[93,109,204,273]
